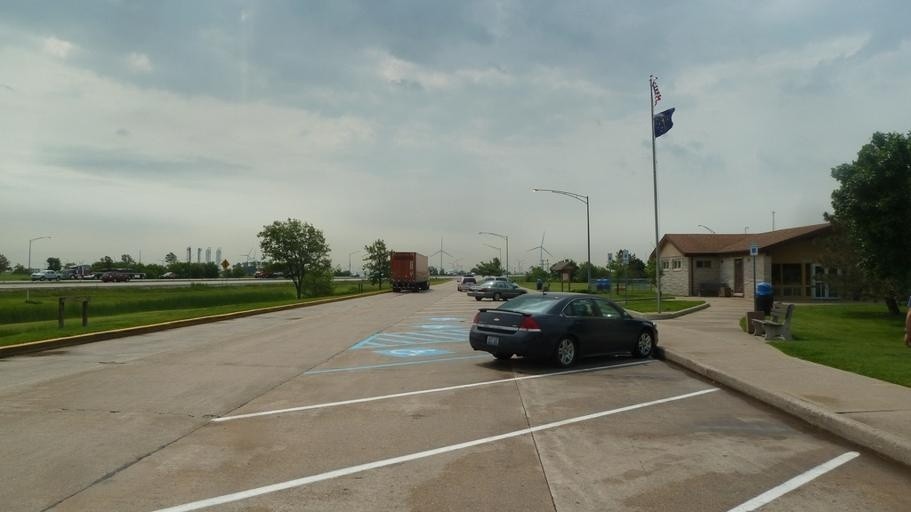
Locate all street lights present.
[349,250,361,275]
[483,243,502,276]
[532,189,593,293]
[29,236,52,276]
[478,232,508,279]
[698,225,717,234]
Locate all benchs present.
[750,298,797,342]
[695,279,724,298]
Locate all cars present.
[351,272,360,278]
[469,291,658,369]
[467,280,528,301]
[83,273,100,280]
[31,269,61,282]
[160,272,176,279]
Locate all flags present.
[653,107,673,138]
[650,73,661,106]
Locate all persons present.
[903,295,911,347]
[535,276,542,290]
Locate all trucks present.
[254,265,280,278]
[390,251,433,292]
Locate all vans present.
[457,275,477,292]
[482,276,508,282]
[101,271,131,282]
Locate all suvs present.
[57,269,78,280]
